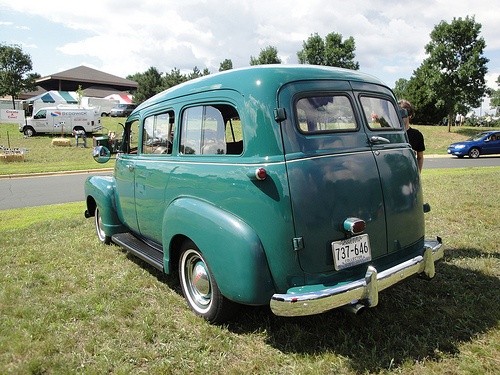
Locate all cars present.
[84,62,446,327]
[447,131,500,159]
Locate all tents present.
[20,90,82,105]
[103,93,145,105]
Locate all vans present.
[19,104,103,138]
[110,103,138,118]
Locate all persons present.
[486,115,492,127]
[489,135,496,141]
[398,100,425,175]
[454,112,464,126]
